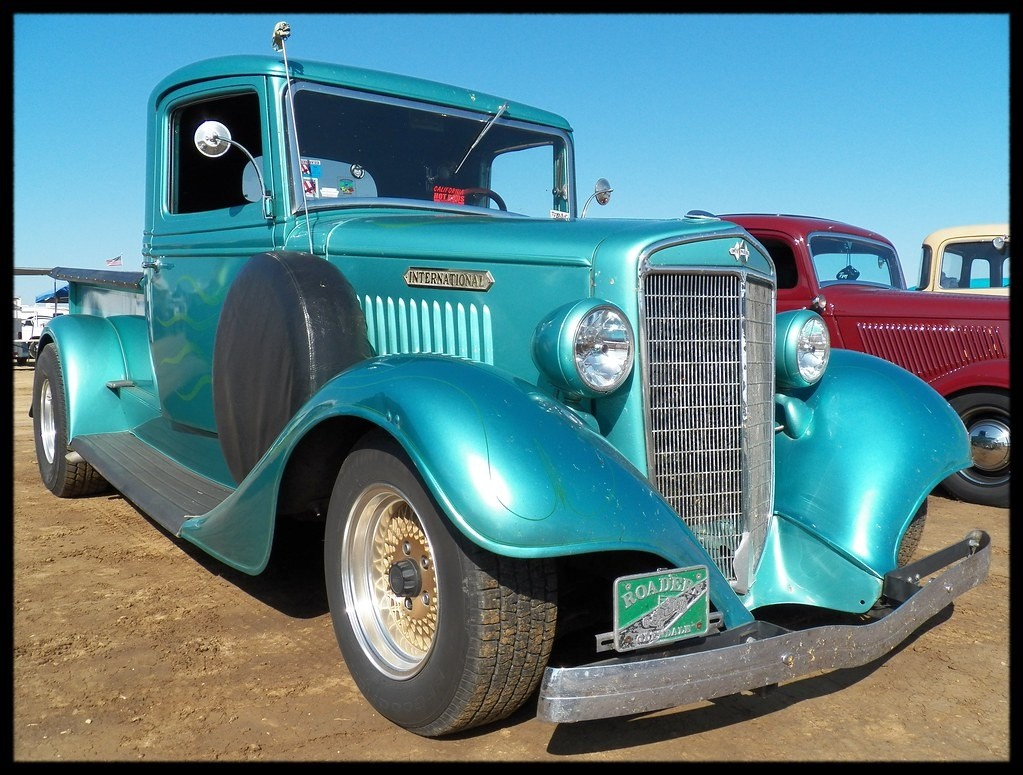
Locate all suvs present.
[717,213,1011,508]
[31,21,991,741]
